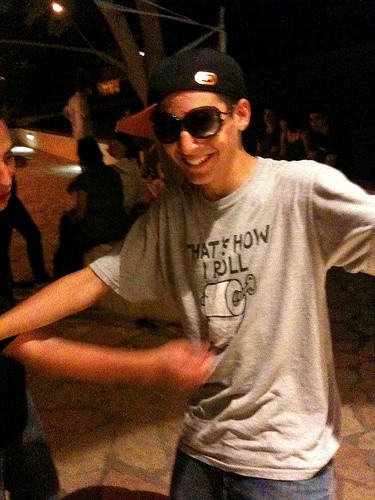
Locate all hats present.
[147,47,245,106]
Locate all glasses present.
[147,103,237,144]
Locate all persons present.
[1,47,375,500]
[52,134,125,282]
[0,115,221,500]
